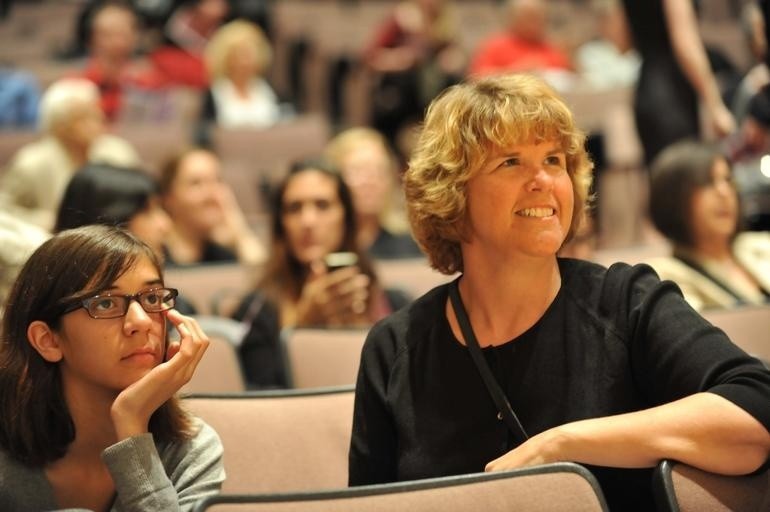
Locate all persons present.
[346,71,770,509]
[0,224,228,511]
[0,0,769,389]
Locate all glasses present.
[64,286,178,319]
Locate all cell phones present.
[326,253,361,270]
